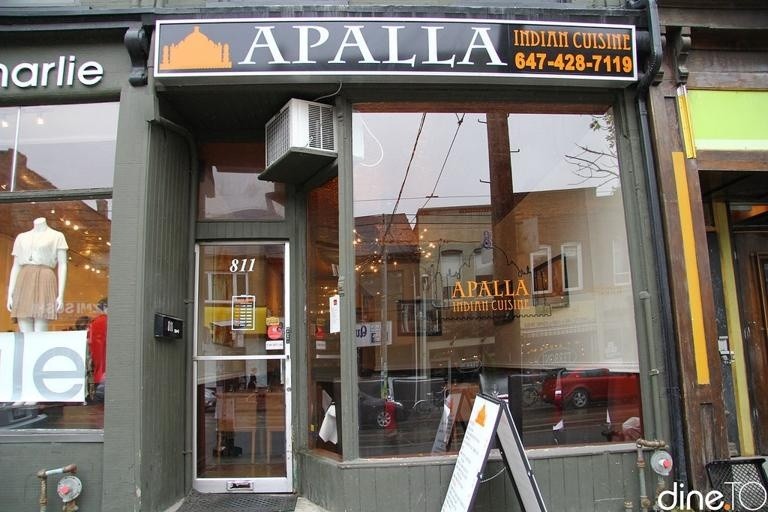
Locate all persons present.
[7,217,72,408]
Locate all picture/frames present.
[397,298,443,337]
[528,242,556,298]
[558,240,584,296]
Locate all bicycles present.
[413,385,448,420]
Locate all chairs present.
[214,392,258,464]
[264,391,285,464]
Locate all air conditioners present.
[262,97,365,172]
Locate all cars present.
[540,367,641,409]
[358,392,408,429]
